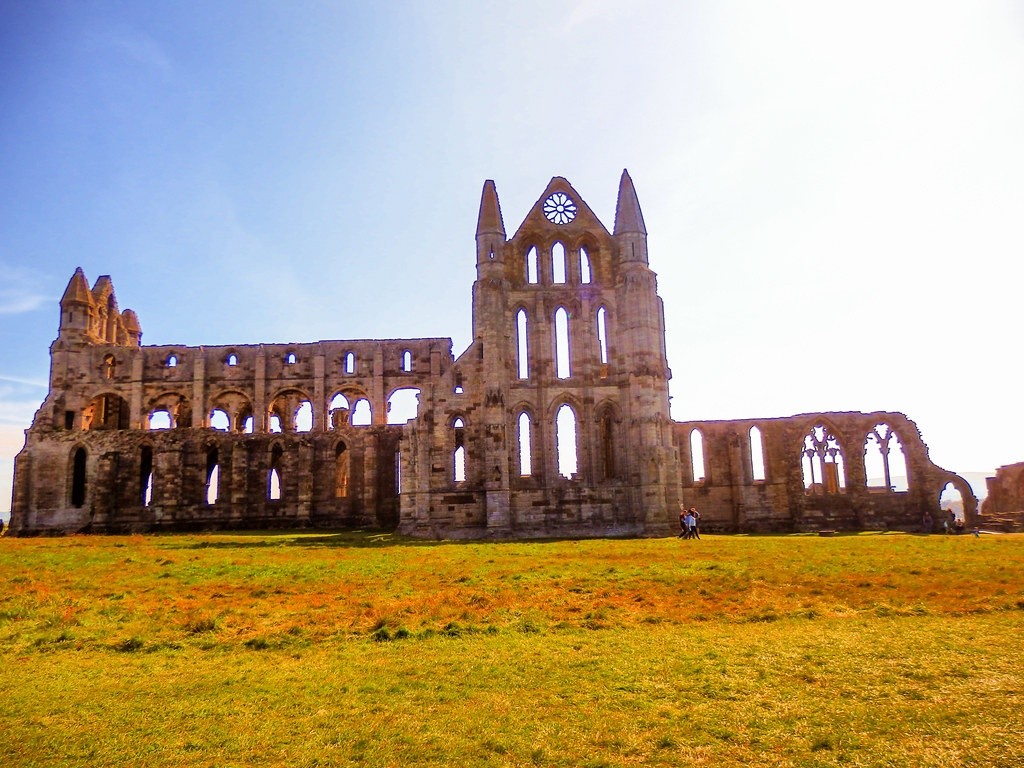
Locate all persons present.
[974,527,980,538]
[922,511,934,533]
[943,517,949,535]
[677,507,701,540]
